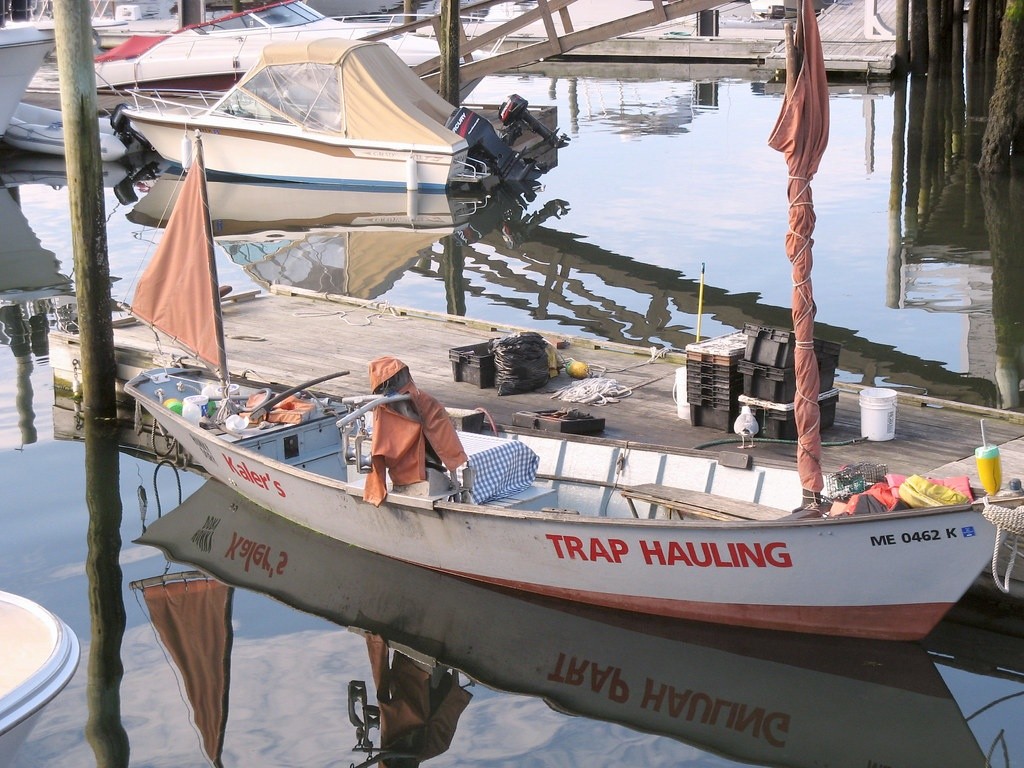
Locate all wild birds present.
[734,405,759,449]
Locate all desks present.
[366,426,540,505]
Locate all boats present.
[115,0,1024,644]
[118,36,572,189]
[127,477,994,768]
[0,0,493,160]
[122,163,570,300]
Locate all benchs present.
[621,483,791,522]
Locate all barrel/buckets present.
[184,395,209,417]
[163,398,183,416]
[673,367,691,419]
[202,384,240,410]
[859,388,899,441]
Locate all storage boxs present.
[448,342,495,389]
[685,322,842,440]
